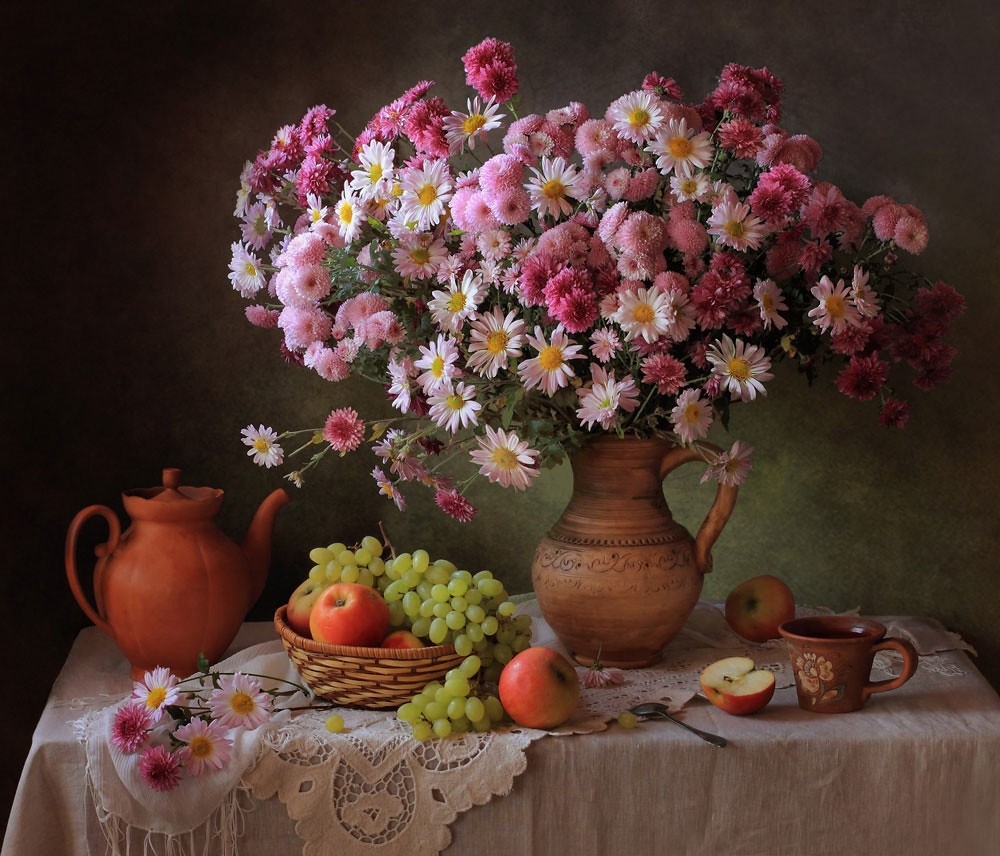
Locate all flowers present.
[110,649,373,792]
[228,32,962,522]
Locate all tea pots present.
[62,467,289,684]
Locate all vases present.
[530,428,743,670]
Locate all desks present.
[0,618,1000,856]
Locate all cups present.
[779,614,918,712]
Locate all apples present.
[725,575,795,639]
[310,583,388,649]
[700,657,775,715]
[499,646,579,729]
[382,630,425,649]
[287,578,323,634]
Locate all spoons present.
[631,702,730,750]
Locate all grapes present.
[618,712,637,728]
[309,536,533,739]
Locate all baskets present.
[274,603,462,711]
